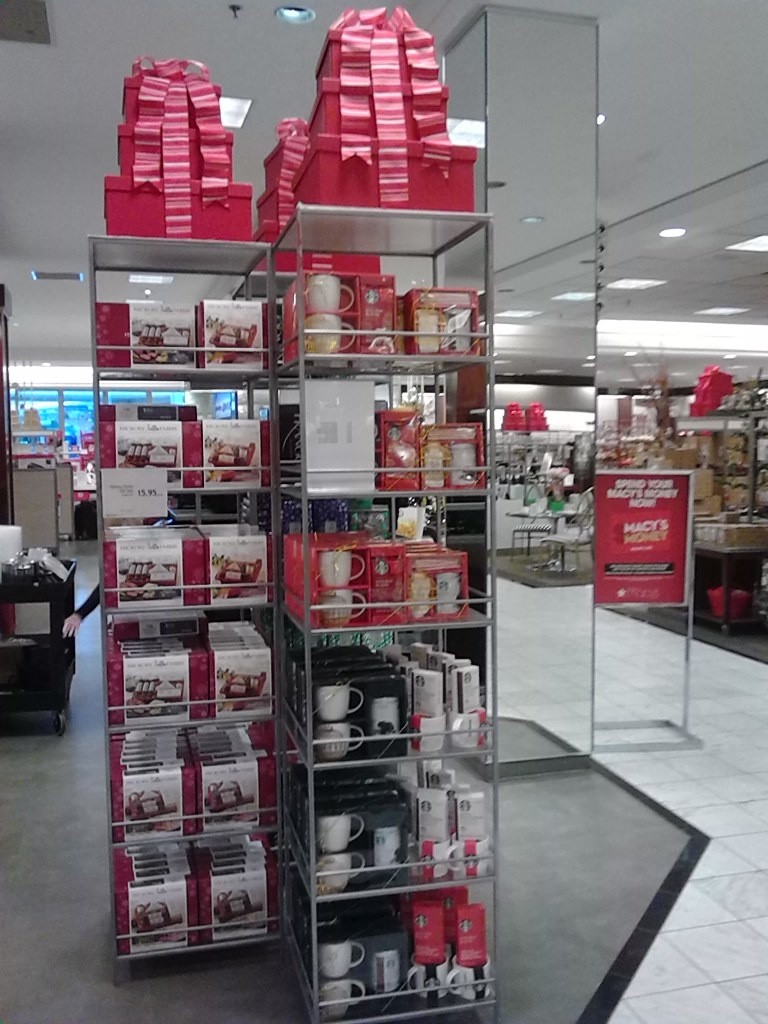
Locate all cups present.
[412,712,446,752]
[409,574,433,617]
[369,952,401,996]
[319,856,364,893]
[317,684,362,724]
[444,832,490,877]
[374,824,400,872]
[438,574,460,615]
[321,814,365,854]
[322,942,364,977]
[447,309,471,352]
[371,695,401,741]
[418,836,450,881]
[320,590,366,627]
[418,317,437,354]
[322,982,364,1018]
[319,553,365,585]
[450,443,477,485]
[312,724,363,759]
[306,315,354,354]
[450,708,486,751]
[423,443,442,488]
[405,954,447,1002]
[305,275,355,312]
[447,958,492,999]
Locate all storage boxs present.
[0,557,79,737]
[101,54,256,242]
[250,6,479,273]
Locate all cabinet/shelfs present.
[597,374,767,639]
[10,378,240,545]
[86,232,291,965]
[224,198,503,1022]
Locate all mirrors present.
[434,4,593,781]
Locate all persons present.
[61,581,100,639]
